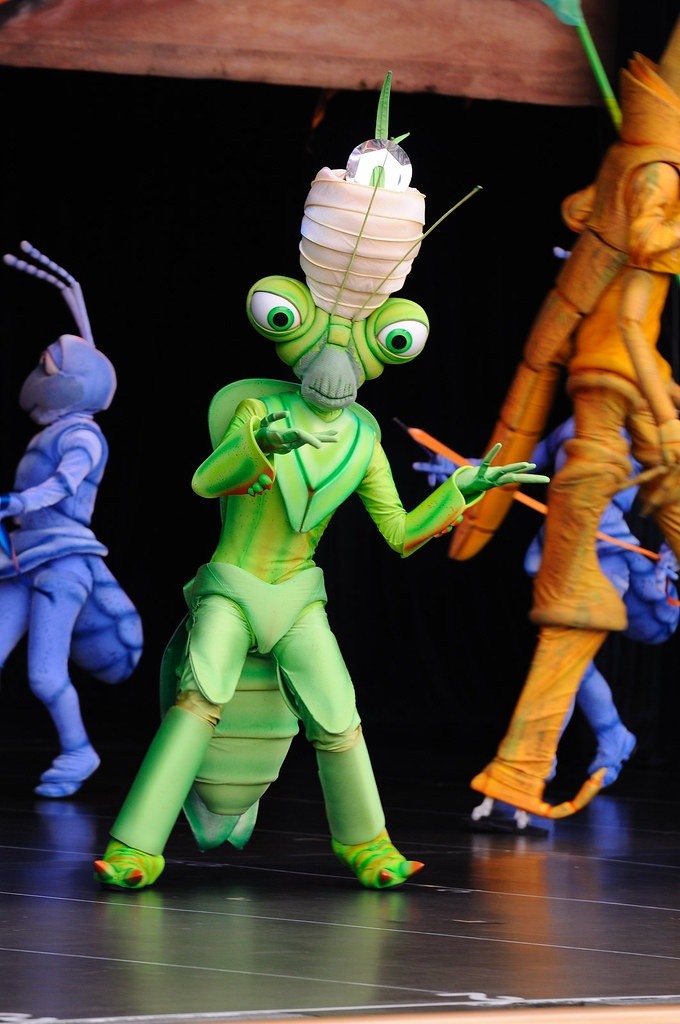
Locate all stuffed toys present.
[0,238,143,799]
[408,18,679,817]
[77,69,549,890]
[411,245,678,832]
